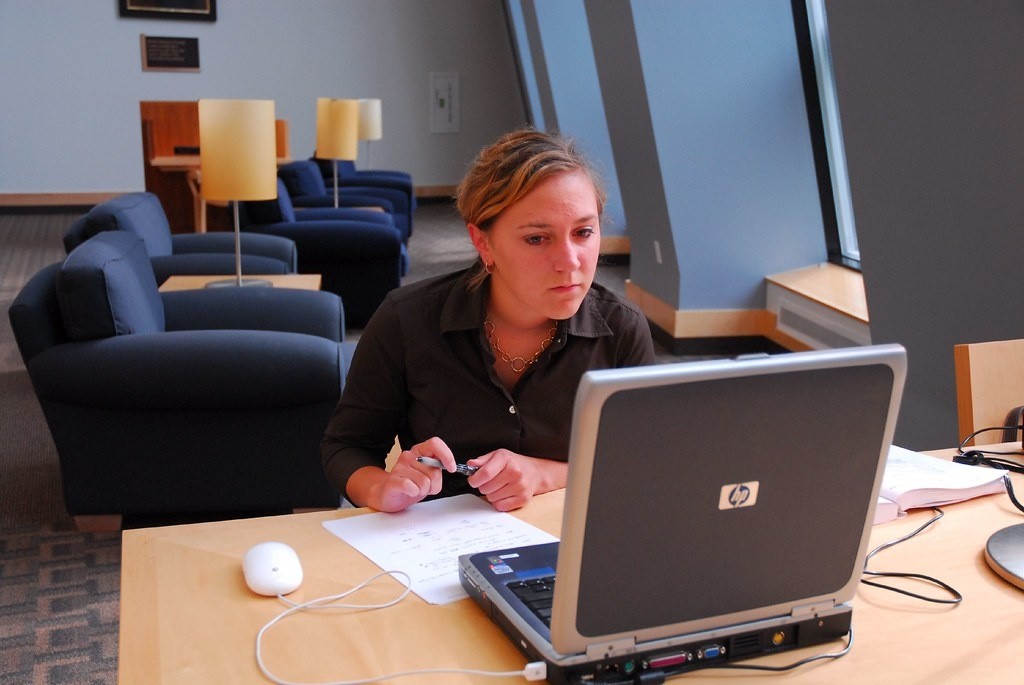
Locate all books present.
[874,442,1009,527]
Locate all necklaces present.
[484,310,560,376]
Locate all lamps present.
[356,97,383,172]
[192,94,284,288]
[312,93,362,207]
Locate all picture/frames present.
[116,0,220,24]
[138,33,204,73]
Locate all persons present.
[314,127,659,515]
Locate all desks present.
[113,438,1024,685]
[156,271,324,294]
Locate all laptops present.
[457,344,907,685]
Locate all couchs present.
[7,227,361,528]
[310,145,419,219]
[276,156,397,225]
[239,172,409,316]
[67,190,299,282]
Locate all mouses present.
[242,543,304,598]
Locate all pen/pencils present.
[416,456,482,477]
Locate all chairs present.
[954,335,1024,450]
